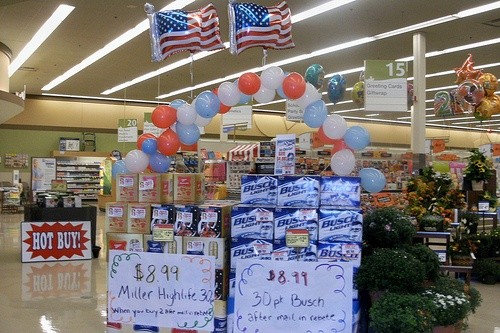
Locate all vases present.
[370,291,386,304]
[433,323,461,333]
[453,255,471,266]
[471,180,483,191]
[482,274,496,284]
[424,227,436,232]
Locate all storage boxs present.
[228,175,364,333]
[115,174,138,202]
[105,200,237,333]
[138,173,173,203]
[174,172,205,202]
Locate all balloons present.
[407,82,413,110]
[144,2,226,62]
[434,54,500,120]
[227,1,296,55]
[352,81,365,105]
[327,74,346,107]
[111,67,387,195]
[359,71,364,81]
[305,63,325,89]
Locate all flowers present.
[355,207,481,333]
[404,147,500,278]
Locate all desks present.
[24,204,97,246]
